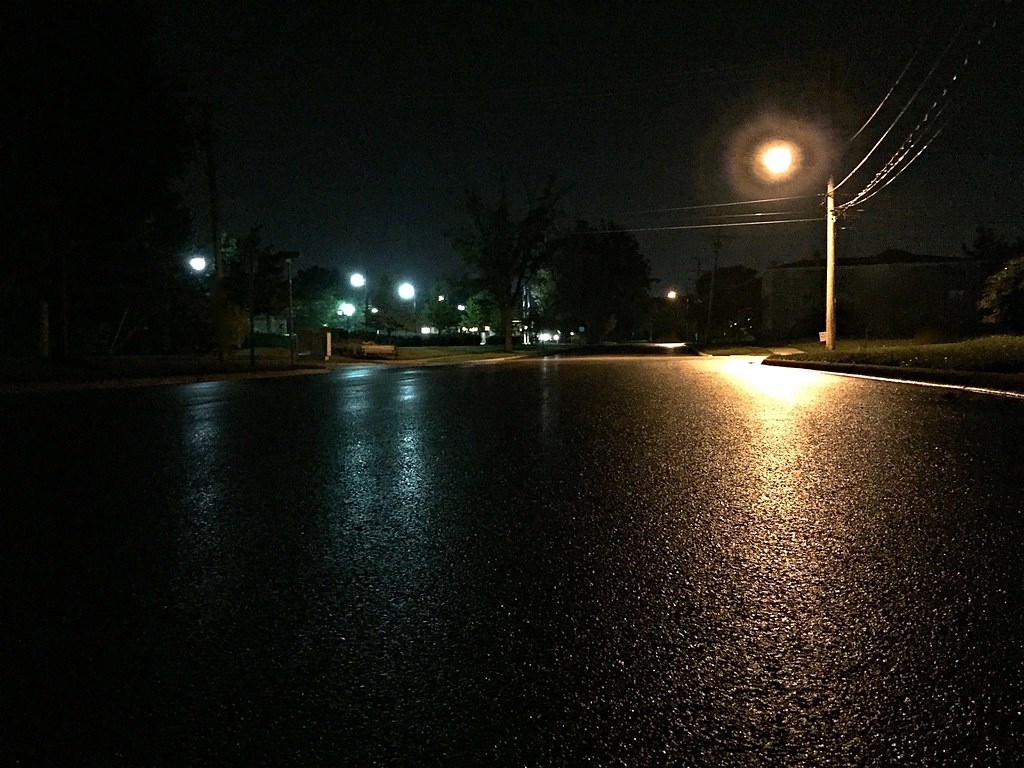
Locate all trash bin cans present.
[312,330,332,361]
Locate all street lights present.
[750,136,833,356]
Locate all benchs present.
[360,344,400,361]
